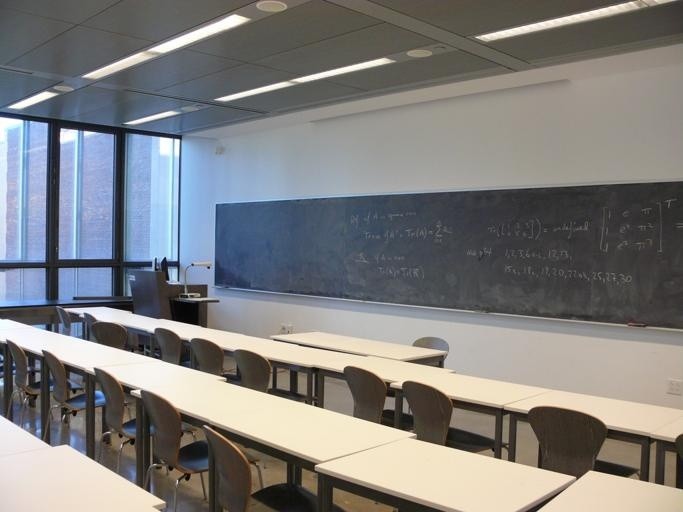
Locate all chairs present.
[409,335,451,370]
[6,339,85,430]
[526,403,640,481]
[140,388,266,512]
[190,337,240,385]
[400,380,512,454]
[202,425,346,512]
[88,322,131,348]
[232,347,319,404]
[41,348,131,441]
[342,364,415,432]
[152,327,185,364]
[53,305,97,337]
[94,366,198,475]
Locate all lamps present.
[180,260,211,298]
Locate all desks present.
[270,330,448,393]
[534,468,683,510]
[503,389,681,461]
[315,438,575,511]
[0,413,166,510]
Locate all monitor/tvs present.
[160,256,169,281]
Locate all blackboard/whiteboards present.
[212,177,683,332]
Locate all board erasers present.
[628,323,648,327]
